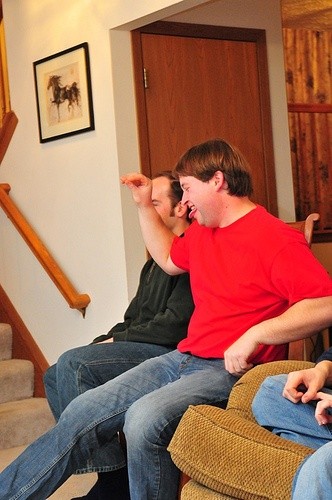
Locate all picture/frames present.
[31,42,96,144]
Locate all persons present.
[42,170,195,500]
[251,348,332,500]
[0,142,332,500]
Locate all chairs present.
[278,212,322,361]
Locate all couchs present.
[166,359,318,500]
